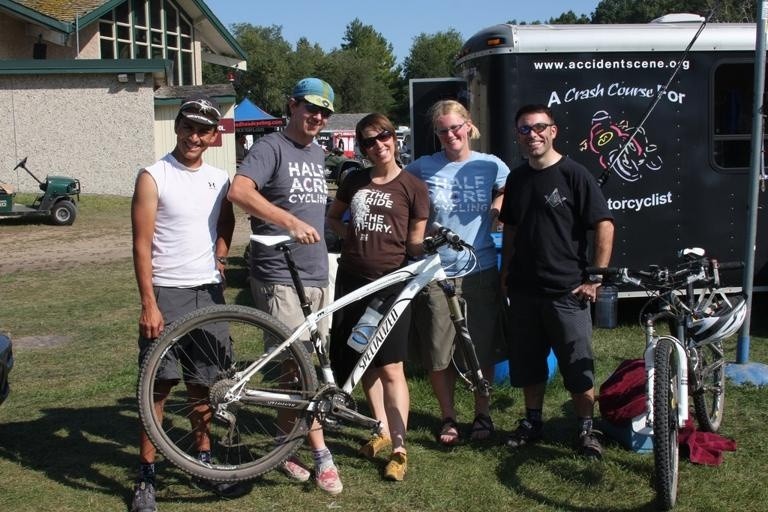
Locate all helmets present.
[687,295,747,344]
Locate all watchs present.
[215,256,227,266]
[583,275,603,285]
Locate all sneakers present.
[362,434,407,481]
[573,432,601,456]
[278,456,343,495]
[505,420,541,447]
[191,461,242,496]
[131,475,157,512]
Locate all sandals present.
[440,414,494,445]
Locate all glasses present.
[305,103,331,117]
[517,123,552,135]
[363,131,391,148]
[435,123,465,135]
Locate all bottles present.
[346,296,388,355]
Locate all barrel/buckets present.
[594,285,619,329]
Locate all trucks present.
[407,13,766,330]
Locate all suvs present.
[314,126,412,192]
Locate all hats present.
[180,98,221,125]
[293,78,335,112]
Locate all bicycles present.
[573,245,748,508]
[133,219,491,486]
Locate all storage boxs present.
[609,412,656,455]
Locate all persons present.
[498,104,617,459]
[326,112,430,482]
[227,77,344,494]
[403,99,511,445]
[129,97,235,512]
[337,134,344,152]
